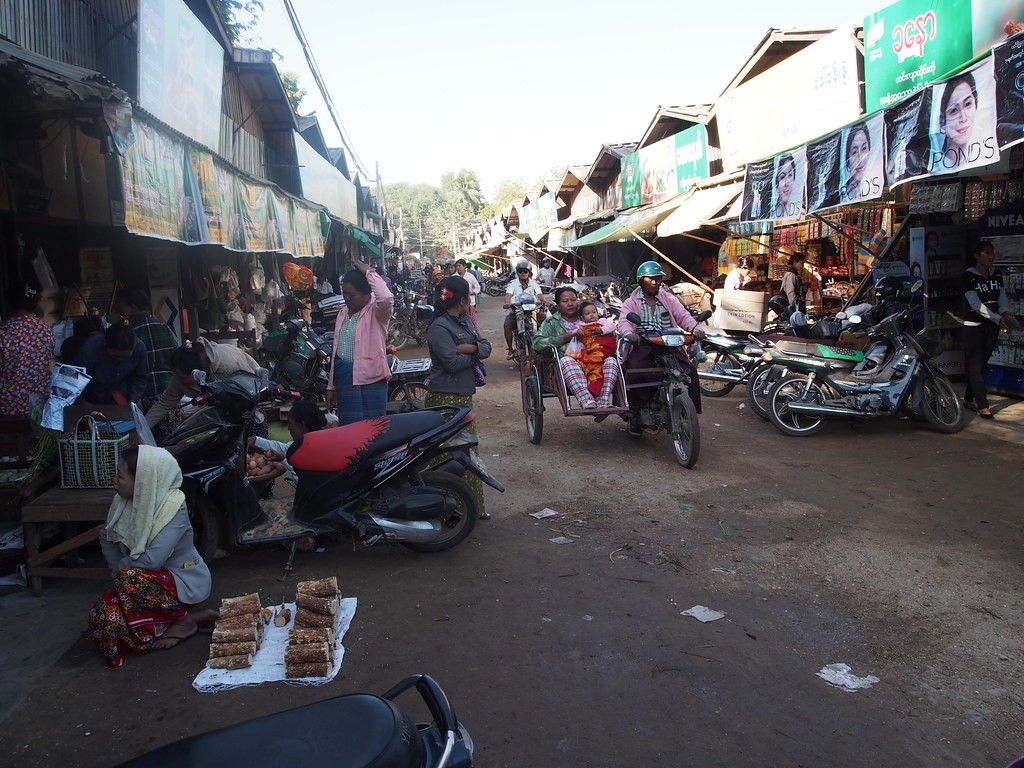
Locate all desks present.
[22,483,119,595]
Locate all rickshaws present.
[520,299,713,467]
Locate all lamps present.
[75,115,120,160]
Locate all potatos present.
[245,450,275,477]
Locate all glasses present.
[518,270,528,273]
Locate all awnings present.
[559,179,792,290]
[468,230,583,275]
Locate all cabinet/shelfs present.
[910,224,970,380]
[819,273,867,317]
[980,205,1024,399]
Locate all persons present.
[779,252,806,304]
[326,257,394,427]
[842,122,870,201]
[532,286,619,423]
[925,231,939,256]
[432,258,485,337]
[89,445,220,669]
[503,259,553,361]
[246,399,334,553]
[773,153,796,218]
[910,261,922,276]
[425,275,492,528]
[724,256,754,290]
[60,285,268,430]
[961,241,1020,419]
[940,72,978,166]
[618,260,705,436]
[0,275,56,431]
[537,257,556,287]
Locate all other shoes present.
[962,402,977,411]
[479,513,491,520]
[623,403,639,418]
[977,409,994,419]
[507,354,513,360]
[585,404,614,423]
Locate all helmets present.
[637,260,666,282]
[445,259,457,266]
[542,257,551,264]
[516,259,533,275]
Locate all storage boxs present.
[712,288,770,334]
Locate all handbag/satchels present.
[563,337,578,359]
[56,411,130,489]
[473,359,487,386]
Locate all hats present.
[433,270,442,275]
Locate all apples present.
[564,348,581,359]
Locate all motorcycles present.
[111,670,479,768]
[746,279,959,439]
[483,271,517,297]
[281,281,433,350]
[578,281,639,299]
[501,291,549,369]
[161,364,510,586]
[257,306,434,415]
[691,295,828,398]
